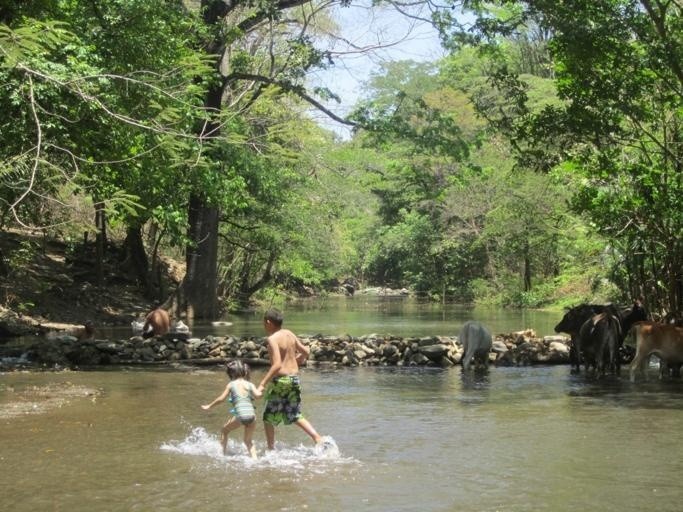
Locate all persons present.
[201,359,265,460]
[257,307,324,453]
[74,319,106,344]
[141,298,170,341]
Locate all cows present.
[142,308,176,339]
[554,298,683,383]
[460,320,492,376]
